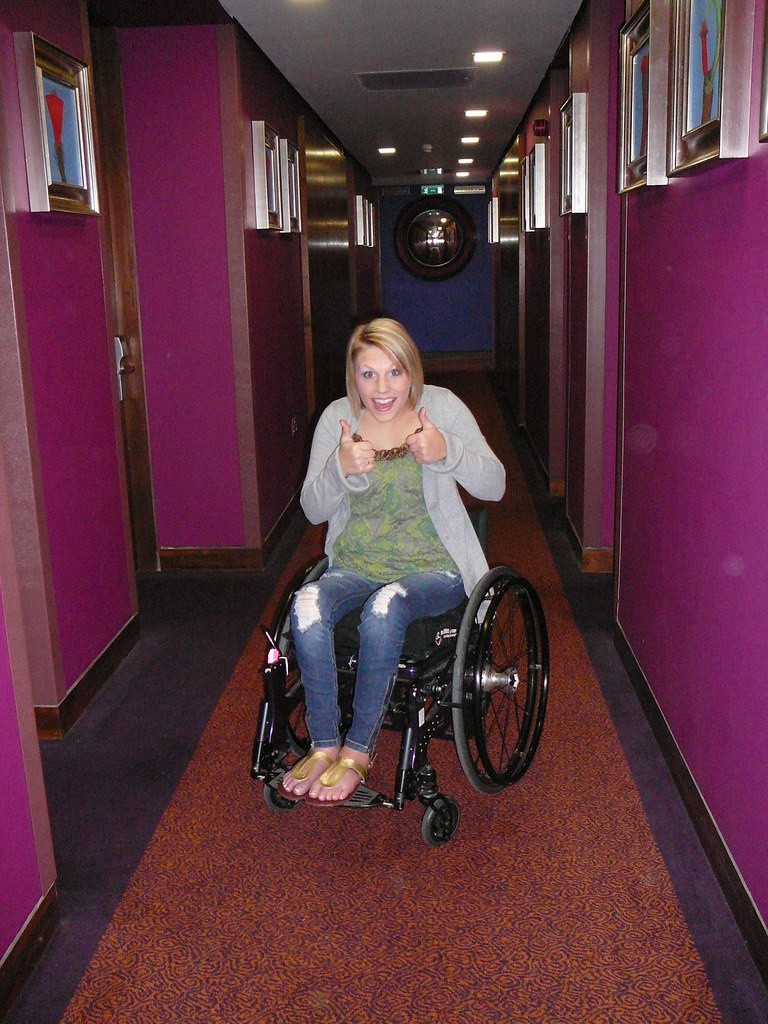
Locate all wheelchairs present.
[250,509,550,846]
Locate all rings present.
[367,459,370,464]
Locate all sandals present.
[278,750,335,801]
[306,756,373,806]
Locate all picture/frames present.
[560,92,588,216]
[13,30,102,219]
[356,195,375,248]
[487,197,498,244]
[615,0,768,198]
[521,143,546,232]
[252,120,303,234]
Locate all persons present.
[281,318,505,800]
